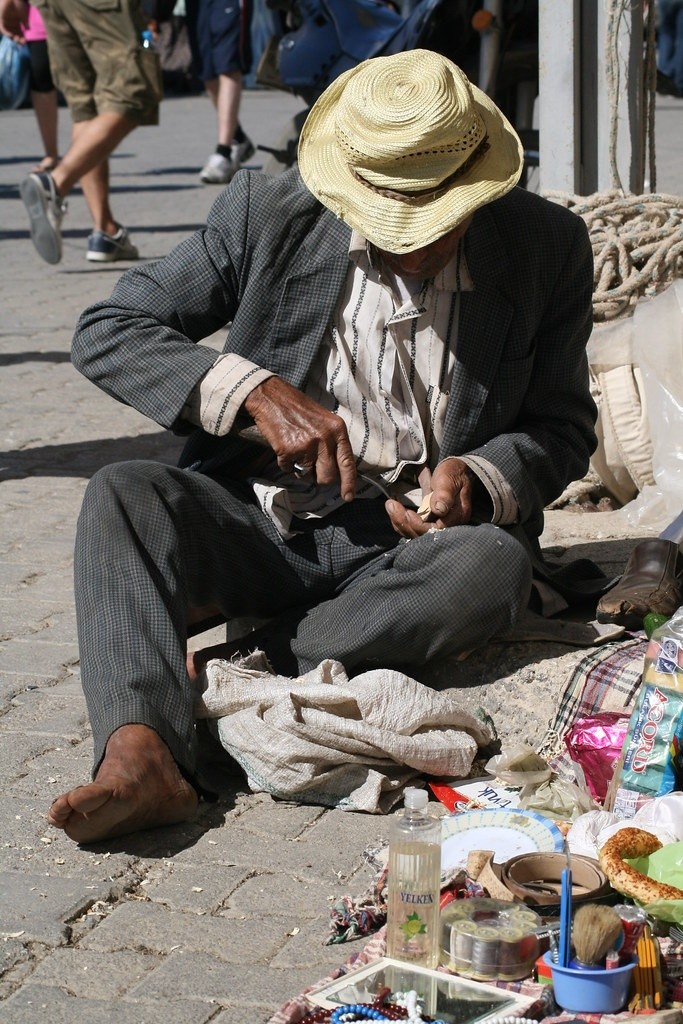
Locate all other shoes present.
[200,153,236,183]
[30,156,60,172]
[229,136,254,172]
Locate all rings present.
[294,463,312,475]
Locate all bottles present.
[142,31,156,49]
[382,789,442,1020]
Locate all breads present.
[599,828,683,905]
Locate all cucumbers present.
[643,613,670,640]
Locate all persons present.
[0,0,162,265]
[147,0,255,183]
[49,50,600,844]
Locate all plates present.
[442,807,565,876]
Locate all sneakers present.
[19,173,67,264]
[86,222,137,262]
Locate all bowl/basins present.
[542,951,640,1015]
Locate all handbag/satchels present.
[0,35,32,112]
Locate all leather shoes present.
[596,538,683,623]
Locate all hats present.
[297,48,523,255]
